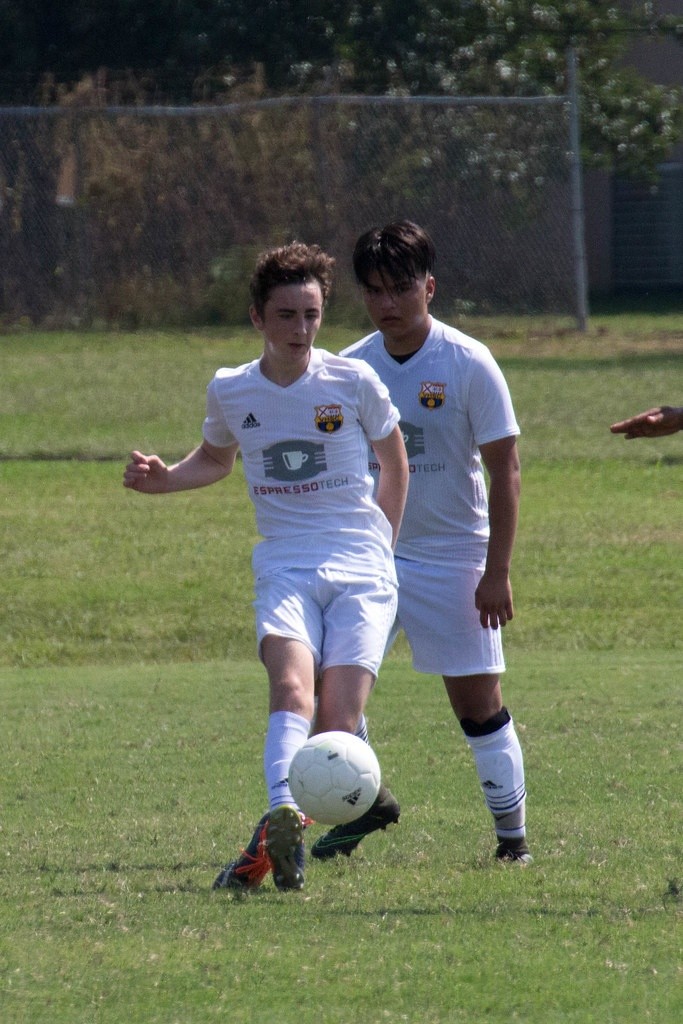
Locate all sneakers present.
[265,805,305,892]
[494,836,534,869]
[213,807,315,895]
[311,789,398,858]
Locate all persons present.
[607,402,683,445]
[328,219,535,866]
[125,241,413,894]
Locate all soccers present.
[288,732,383,827]
